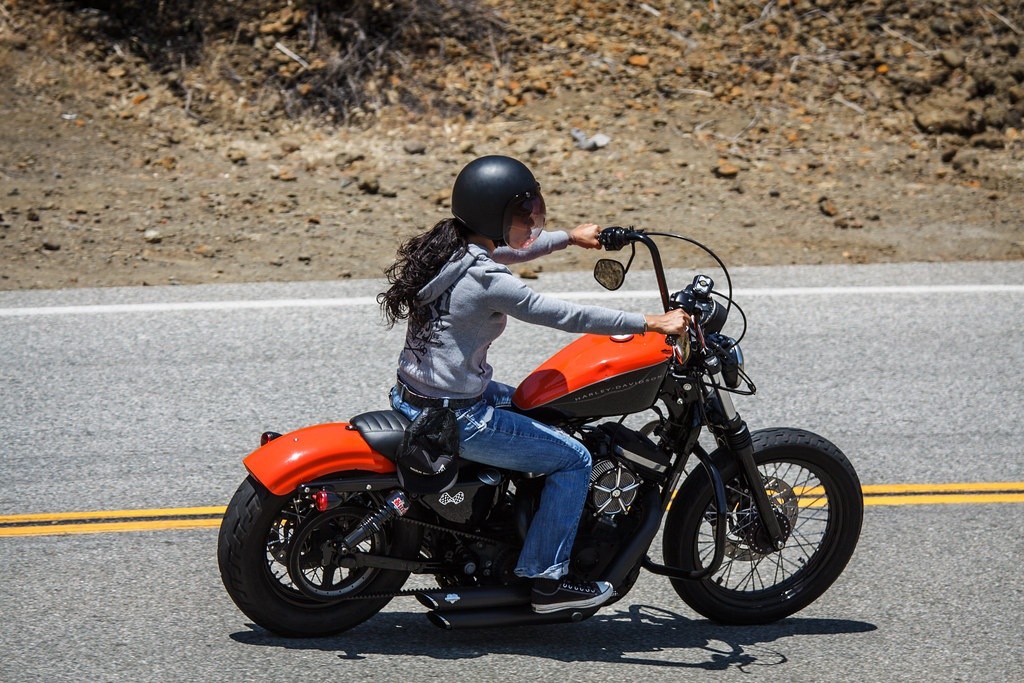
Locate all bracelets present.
[641,315,647,335]
[572,234,576,243]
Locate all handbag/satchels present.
[397,407,461,499]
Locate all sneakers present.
[531,580,613,613]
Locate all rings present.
[683,326,689,332]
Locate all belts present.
[397,378,483,410]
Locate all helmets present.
[451,155,546,252]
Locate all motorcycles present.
[215,223,864,637]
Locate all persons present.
[376,155,692,614]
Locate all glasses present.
[514,209,531,223]
